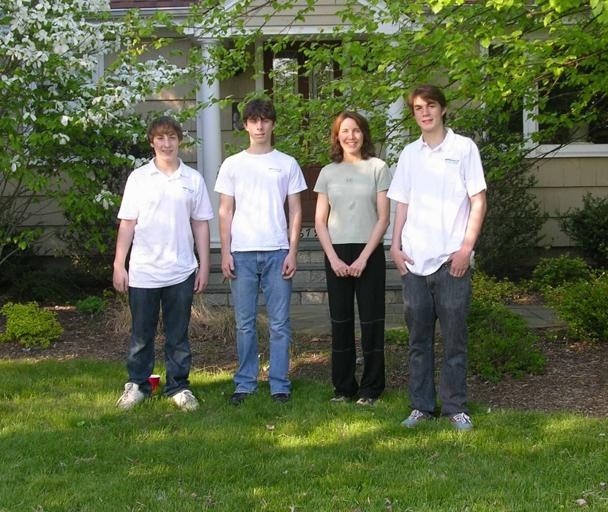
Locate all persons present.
[312,110,393,404]
[112,116,214,413]
[212,97,308,408]
[384,83,487,433]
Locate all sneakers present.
[448,413,474,431]
[402,410,432,429]
[272,393,289,404]
[357,398,378,406]
[116,382,149,409]
[231,393,249,406]
[173,390,200,413]
[331,396,352,403]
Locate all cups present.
[147,374,161,395]
